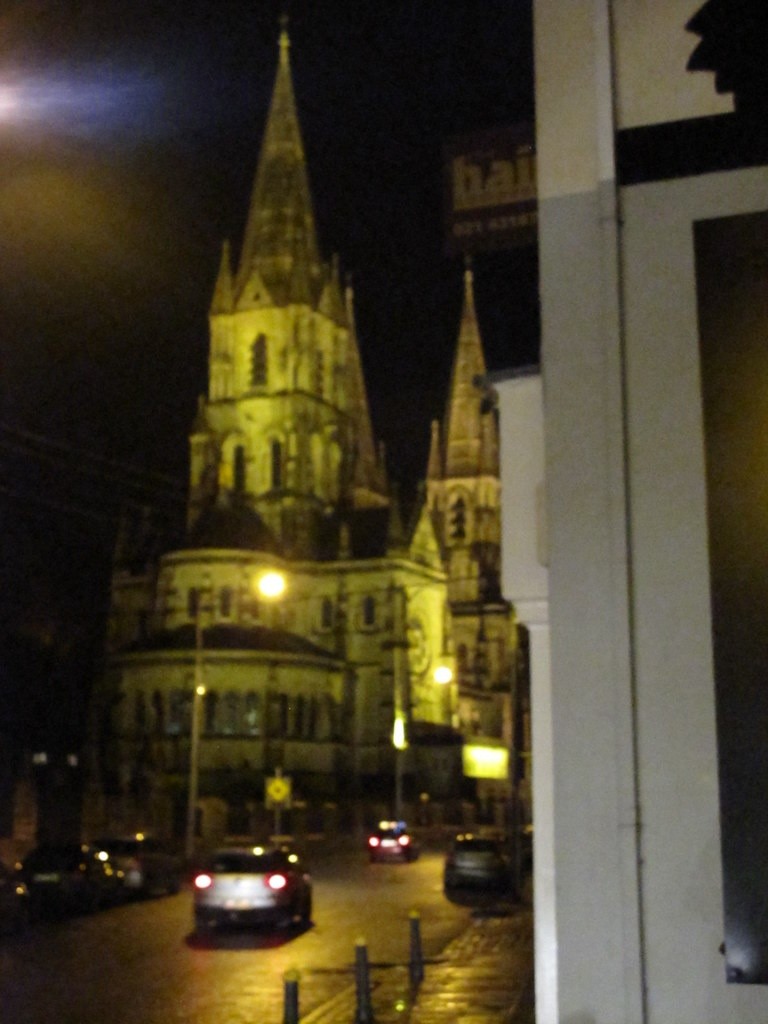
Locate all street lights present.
[185,564,293,870]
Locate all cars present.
[368,820,418,861]
[1,841,179,935]
[443,838,510,899]
[190,843,313,938]
